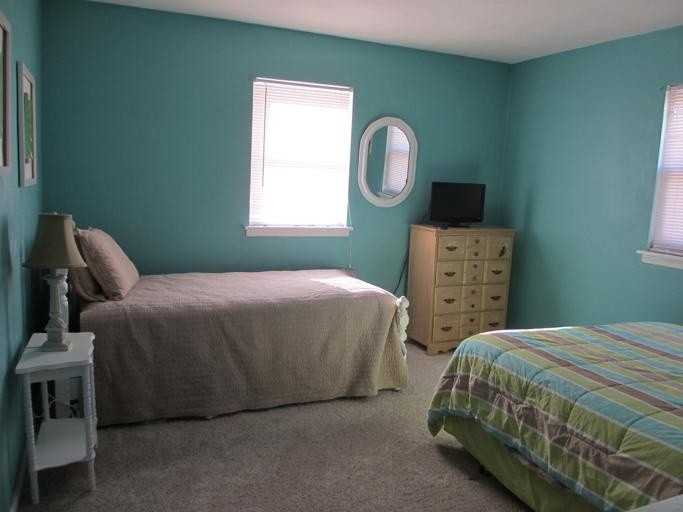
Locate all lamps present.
[22,212,86,351]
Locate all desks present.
[14,330,98,504]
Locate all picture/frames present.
[0,10,39,188]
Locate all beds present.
[427,320,682,512]
[79,269,403,426]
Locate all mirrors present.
[357,117,419,207]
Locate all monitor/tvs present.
[430,182,486,227]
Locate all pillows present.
[63,226,139,301]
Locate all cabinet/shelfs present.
[406,226,515,356]
